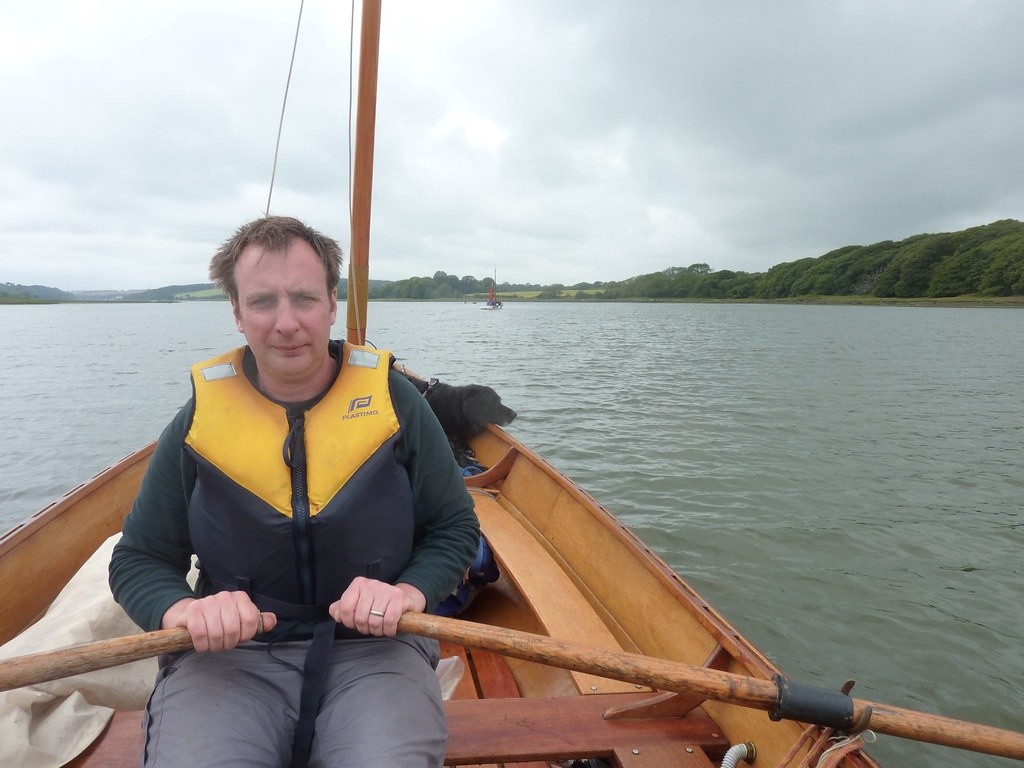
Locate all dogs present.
[396,370,517,468]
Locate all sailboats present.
[480,267,504,310]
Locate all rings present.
[369,609,384,616]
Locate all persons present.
[107,217,480,767]
